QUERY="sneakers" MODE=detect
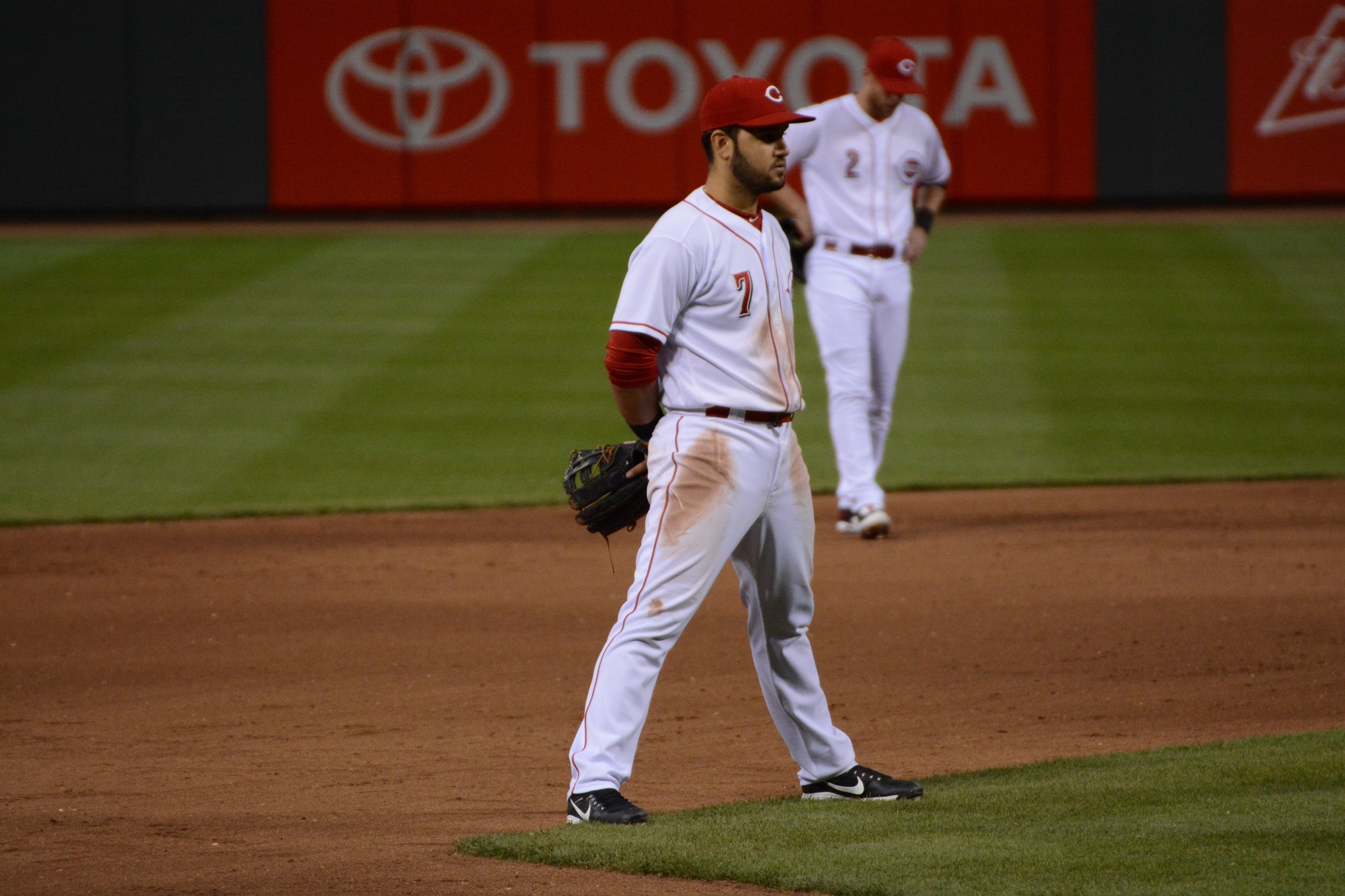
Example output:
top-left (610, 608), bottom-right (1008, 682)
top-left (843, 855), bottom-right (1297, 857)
top-left (837, 509), bottom-right (861, 535)
top-left (859, 504), bottom-right (890, 537)
top-left (801, 765), bottom-right (924, 802)
top-left (566, 788), bottom-right (648, 824)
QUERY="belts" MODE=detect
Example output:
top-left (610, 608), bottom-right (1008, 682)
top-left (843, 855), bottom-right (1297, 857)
top-left (825, 242), bottom-right (895, 259)
top-left (705, 406), bottom-right (793, 427)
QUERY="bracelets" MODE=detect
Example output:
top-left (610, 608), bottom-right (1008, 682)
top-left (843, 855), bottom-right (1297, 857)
top-left (915, 207), bottom-right (933, 232)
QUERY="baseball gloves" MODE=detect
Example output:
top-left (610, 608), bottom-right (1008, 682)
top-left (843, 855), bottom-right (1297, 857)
top-left (776, 217), bottom-right (812, 282)
top-left (562, 439), bottom-right (651, 537)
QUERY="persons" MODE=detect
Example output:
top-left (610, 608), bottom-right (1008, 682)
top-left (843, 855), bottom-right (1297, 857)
top-left (565, 78), bottom-right (924, 825)
top-left (765, 37), bottom-right (953, 535)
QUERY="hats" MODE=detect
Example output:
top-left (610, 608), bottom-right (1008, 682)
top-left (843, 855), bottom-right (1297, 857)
top-left (701, 75), bottom-right (816, 131)
top-left (868, 38), bottom-right (926, 95)
